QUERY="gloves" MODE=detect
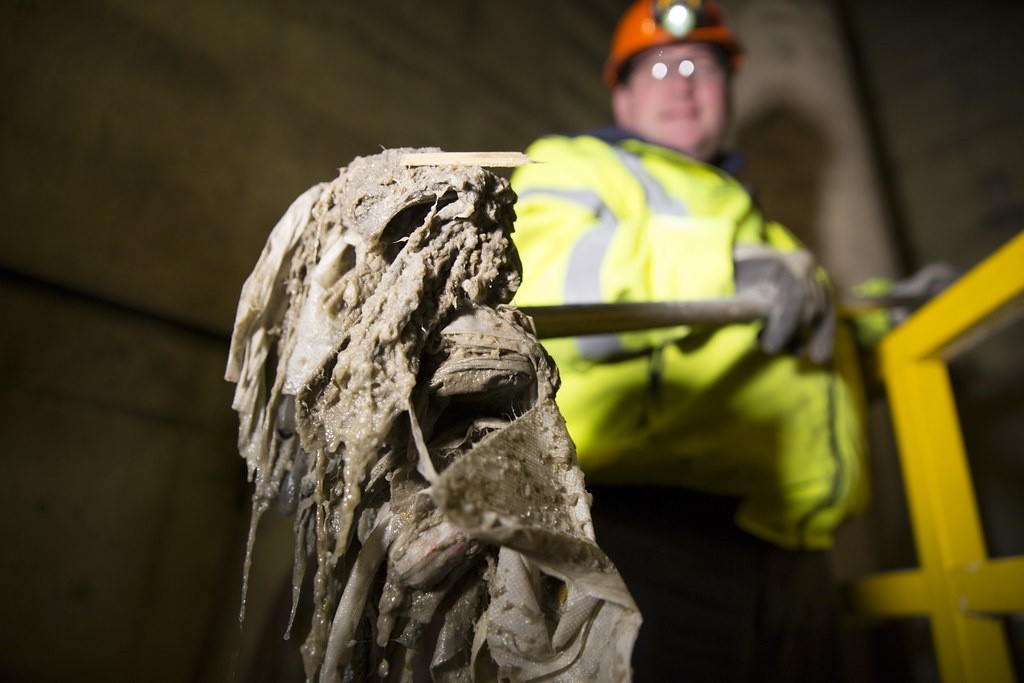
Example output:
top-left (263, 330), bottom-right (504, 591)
top-left (891, 262), bottom-right (954, 322)
top-left (734, 250), bottom-right (838, 365)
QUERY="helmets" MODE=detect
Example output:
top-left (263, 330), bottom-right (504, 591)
top-left (603, 0), bottom-right (746, 90)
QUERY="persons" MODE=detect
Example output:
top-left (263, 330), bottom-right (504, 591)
top-left (503, 1), bottom-right (963, 683)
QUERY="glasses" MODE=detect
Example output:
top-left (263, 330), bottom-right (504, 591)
top-left (631, 56), bottom-right (725, 82)
